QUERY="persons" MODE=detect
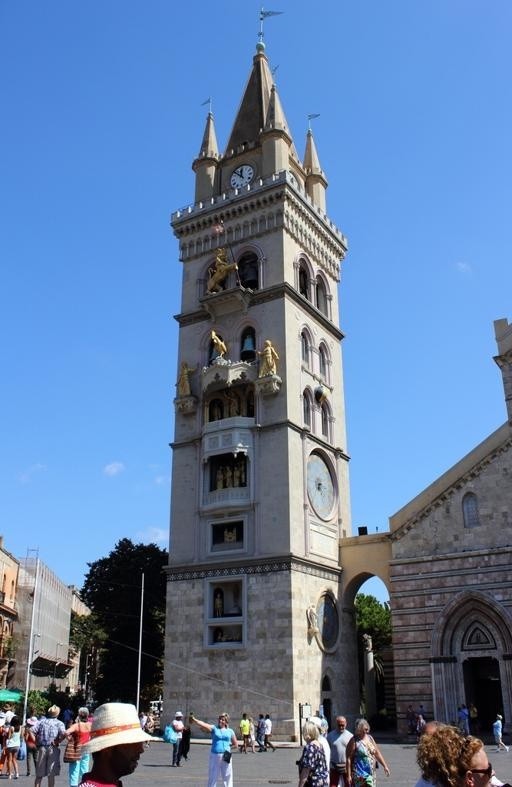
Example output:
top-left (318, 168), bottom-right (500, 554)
top-left (416, 725), bottom-right (511, 786)
top-left (457, 707), bottom-right (462, 726)
top-left (327, 716), bottom-right (355, 787)
top-left (190, 711), bottom-right (238, 787)
top-left (310, 716), bottom-right (332, 785)
top-left (309, 603), bottom-right (319, 628)
top-left (174, 361), bottom-right (199, 397)
top-left (491, 714), bottom-right (509, 752)
top-left (416, 715), bottom-right (427, 736)
top-left (2, 700), bottom-right (92, 787)
top-left (215, 593), bottom-right (222, 617)
top-left (216, 461), bottom-right (245, 489)
top-left (418, 705), bottom-right (425, 717)
top-left (408, 704), bottom-right (415, 727)
top-left (239, 713), bottom-right (276, 754)
top-left (140, 711), bottom-right (147, 728)
top-left (345, 718), bottom-right (390, 787)
top-left (414, 720), bottom-right (450, 787)
top-left (460, 703), bottom-right (470, 733)
top-left (293, 722), bottom-right (330, 786)
top-left (168, 711), bottom-right (188, 767)
top-left (78, 701), bottom-right (165, 786)
top-left (256, 340), bottom-right (278, 379)
top-left (468, 703), bottom-right (478, 732)
top-left (143, 716), bottom-right (155, 748)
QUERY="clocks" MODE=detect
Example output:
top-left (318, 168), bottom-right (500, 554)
top-left (229, 163), bottom-right (254, 188)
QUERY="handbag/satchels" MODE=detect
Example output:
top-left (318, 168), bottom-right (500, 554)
top-left (63, 735), bottom-right (82, 762)
top-left (5, 733), bottom-right (21, 749)
top-left (163, 724), bottom-right (178, 743)
top-left (224, 750), bottom-right (231, 763)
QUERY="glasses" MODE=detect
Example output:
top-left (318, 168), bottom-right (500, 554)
top-left (471, 762), bottom-right (493, 779)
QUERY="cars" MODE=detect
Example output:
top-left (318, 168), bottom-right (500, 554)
top-left (146, 701), bottom-right (164, 715)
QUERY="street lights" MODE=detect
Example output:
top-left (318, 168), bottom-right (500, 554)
top-left (51, 641), bottom-right (68, 707)
top-left (84, 653), bottom-right (95, 705)
top-left (21, 633), bottom-right (42, 742)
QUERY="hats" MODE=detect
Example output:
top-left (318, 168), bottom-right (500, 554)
top-left (3, 703), bottom-right (12, 710)
top-left (25, 704), bottom-right (61, 725)
top-left (78, 700), bottom-right (165, 755)
top-left (174, 712), bottom-right (183, 718)
